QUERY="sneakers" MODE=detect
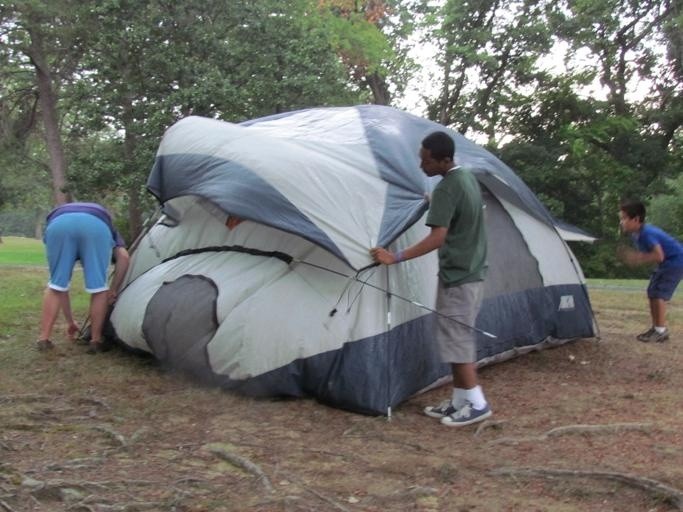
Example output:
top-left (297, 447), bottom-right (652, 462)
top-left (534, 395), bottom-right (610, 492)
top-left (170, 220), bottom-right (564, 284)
top-left (637, 328), bottom-right (668, 342)
top-left (89, 338), bottom-right (112, 353)
top-left (422, 399), bottom-right (456, 419)
top-left (37, 338), bottom-right (55, 350)
top-left (441, 399), bottom-right (493, 426)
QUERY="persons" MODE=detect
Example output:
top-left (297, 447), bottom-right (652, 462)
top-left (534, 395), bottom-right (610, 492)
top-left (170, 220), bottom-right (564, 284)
top-left (369, 129), bottom-right (496, 428)
top-left (38, 200), bottom-right (130, 353)
top-left (616, 198), bottom-right (683, 343)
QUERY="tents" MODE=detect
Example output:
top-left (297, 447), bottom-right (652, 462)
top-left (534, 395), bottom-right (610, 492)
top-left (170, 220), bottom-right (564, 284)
top-left (76, 104), bottom-right (604, 414)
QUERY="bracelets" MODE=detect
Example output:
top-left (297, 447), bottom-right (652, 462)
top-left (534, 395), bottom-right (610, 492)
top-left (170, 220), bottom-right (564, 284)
top-left (394, 250), bottom-right (402, 263)
